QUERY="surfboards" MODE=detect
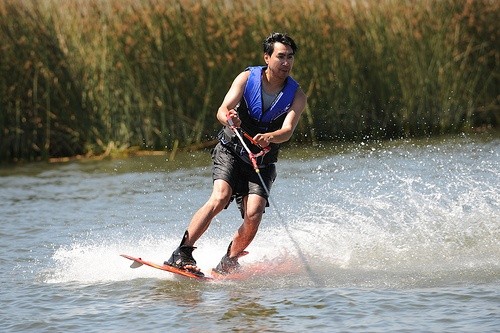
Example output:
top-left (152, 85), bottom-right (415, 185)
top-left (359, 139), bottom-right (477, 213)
top-left (123, 252), bottom-right (258, 282)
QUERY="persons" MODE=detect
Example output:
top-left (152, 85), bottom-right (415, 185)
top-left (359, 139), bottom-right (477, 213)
top-left (163, 32), bottom-right (307, 278)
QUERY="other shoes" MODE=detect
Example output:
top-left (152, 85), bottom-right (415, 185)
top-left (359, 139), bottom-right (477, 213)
top-left (164, 230), bottom-right (205, 278)
top-left (216, 242), bottom-right (249, 278)
